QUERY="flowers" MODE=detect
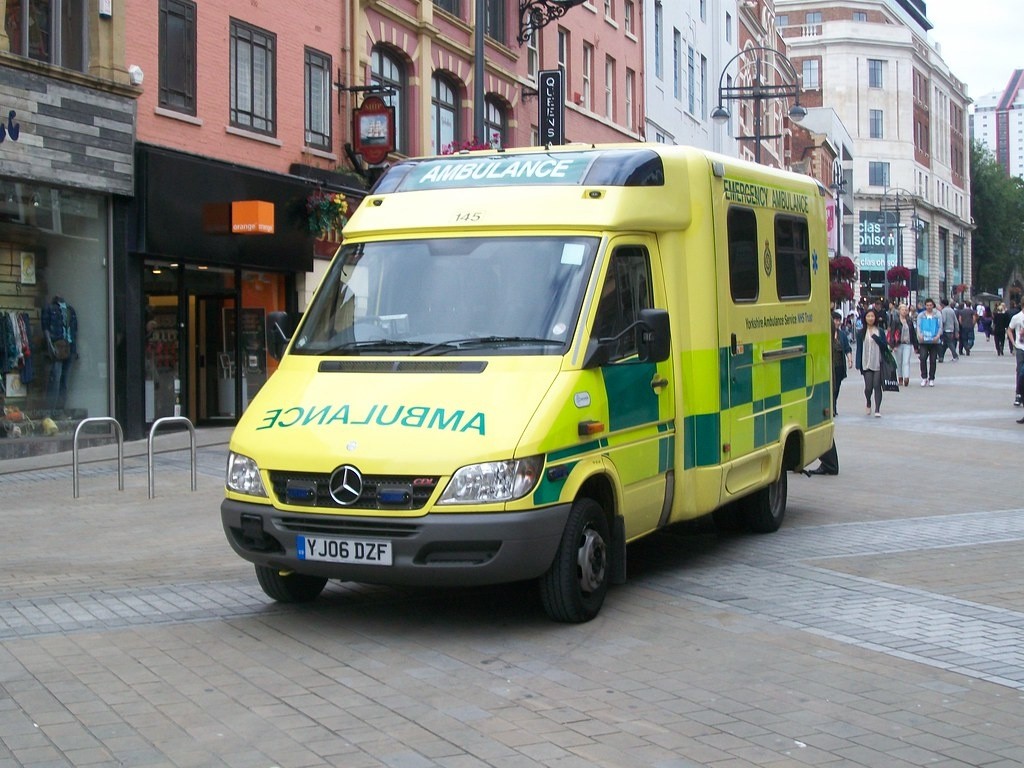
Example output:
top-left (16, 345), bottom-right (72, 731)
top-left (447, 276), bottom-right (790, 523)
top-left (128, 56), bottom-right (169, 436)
top-left (307, 190), bottom-right (348, 235)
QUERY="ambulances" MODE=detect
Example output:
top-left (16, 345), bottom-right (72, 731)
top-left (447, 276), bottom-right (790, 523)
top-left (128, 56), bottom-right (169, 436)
top-left (219, 139), bottom-right (834, 623)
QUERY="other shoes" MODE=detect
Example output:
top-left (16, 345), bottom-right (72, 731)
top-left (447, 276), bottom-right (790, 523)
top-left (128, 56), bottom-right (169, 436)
top-left (952, 357), bottom-right (959, 363)
top-left (866, 407), bottom-right (871, 415)
top-left (965, 346), bottom-right (970, 356)
top-left (874, 413), bottom-right (881, 417)
top-left (1016, 416), bottom-right (1024, 423)
top-left (904, 377), bottom-right (909, 386)
top-left (809, 463), bottom-right (839, 475)
top-left (928, 379), bottom-right (934, 386)
top-left (898, 377), bottom-right (903, 385)
top-left (997, 349), bottom-right (1003, 356)
top-left (938, 357), bottom-right (943, 363)
top-left (920, 378), bottom-right (927, 387)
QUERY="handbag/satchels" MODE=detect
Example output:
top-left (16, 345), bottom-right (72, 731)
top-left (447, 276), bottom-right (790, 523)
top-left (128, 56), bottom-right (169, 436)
top-left (47, 339), bottom-right (71, 363)
top-left (880, 351), bottom-right (899, 392)
top-left (919, 318), bottom-right (941, 344)
top-left (886, 328), bottom-right (900, 346)
top-left (978, 320), bottom-right (985, 332)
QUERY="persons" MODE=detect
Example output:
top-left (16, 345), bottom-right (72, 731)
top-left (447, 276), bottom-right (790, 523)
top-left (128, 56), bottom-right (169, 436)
top-left (830, 296), bottom-right (1024, 424)
top-left (41, 295), bottom-right (78, 419)
top-left (593, 270), bottom-right (619, 338)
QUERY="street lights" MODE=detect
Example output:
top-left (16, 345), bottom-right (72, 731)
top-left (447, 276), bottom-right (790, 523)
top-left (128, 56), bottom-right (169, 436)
top-left (953, 226), bottom-right (968, 300)
top-left (828, 160), bottom-right (848, 256)
top-left (878, 188), bottom-right (918, 266)
top-left (709, 47), bottom-right (808, 164)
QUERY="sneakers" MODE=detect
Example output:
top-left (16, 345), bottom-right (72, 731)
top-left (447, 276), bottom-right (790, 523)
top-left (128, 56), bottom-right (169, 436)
top-left (1014, 393), bottom-right (1022, 405)
